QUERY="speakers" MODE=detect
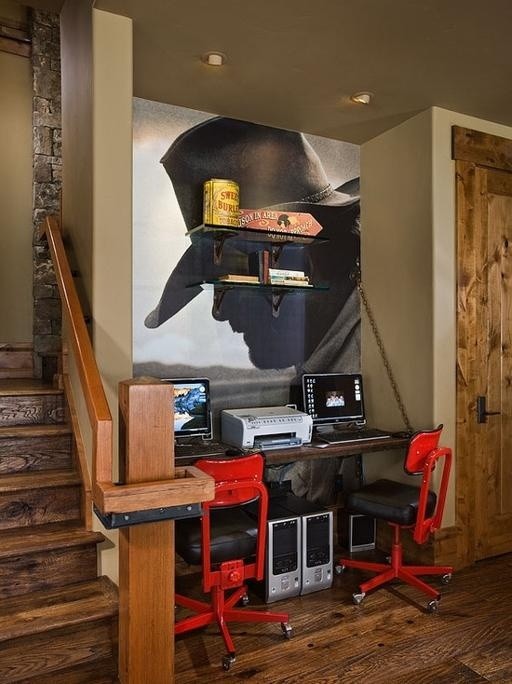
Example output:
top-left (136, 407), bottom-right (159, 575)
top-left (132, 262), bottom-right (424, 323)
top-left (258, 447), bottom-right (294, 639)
top-left (337, 508), bottom-right (377, 553)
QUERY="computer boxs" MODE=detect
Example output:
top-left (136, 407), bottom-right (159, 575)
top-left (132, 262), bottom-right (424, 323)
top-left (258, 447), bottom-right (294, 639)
top-left (241, 494), bottom-right (333, 604)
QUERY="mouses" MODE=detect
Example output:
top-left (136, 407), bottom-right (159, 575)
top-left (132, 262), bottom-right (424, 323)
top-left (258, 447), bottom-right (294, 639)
top-left (392, 431), bottom-right (410, 439)
top-left (224, 448), bottom-right (240, 456)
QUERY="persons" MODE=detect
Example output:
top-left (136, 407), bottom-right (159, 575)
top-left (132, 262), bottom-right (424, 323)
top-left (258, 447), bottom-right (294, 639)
top-left (326, 394), bottom-right (345, 407)
top-left (144, 116), bottom-right (360, 412)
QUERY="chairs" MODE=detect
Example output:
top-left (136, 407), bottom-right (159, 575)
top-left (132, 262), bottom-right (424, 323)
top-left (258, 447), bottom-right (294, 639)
top-left (172, 453), bottom-right (292, 673)
top-left (334, 424), bottom-right (463, 615)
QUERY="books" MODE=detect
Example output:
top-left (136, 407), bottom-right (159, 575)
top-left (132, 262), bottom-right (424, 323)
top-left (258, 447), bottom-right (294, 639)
top-left (218, 250), bottom-right (314, 287)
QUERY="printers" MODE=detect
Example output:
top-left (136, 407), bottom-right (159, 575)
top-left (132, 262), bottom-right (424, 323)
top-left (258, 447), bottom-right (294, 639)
top-left (220, 406), bottom-right (313, 450)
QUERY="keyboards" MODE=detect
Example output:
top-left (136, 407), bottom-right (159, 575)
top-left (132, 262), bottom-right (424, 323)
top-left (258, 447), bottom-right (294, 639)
top-left (317, 428), bottom-right (391, 444)
top-left (174, 444), bottom-right (225, 459)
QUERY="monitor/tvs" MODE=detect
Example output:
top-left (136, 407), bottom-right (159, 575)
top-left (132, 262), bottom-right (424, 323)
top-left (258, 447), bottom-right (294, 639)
top-left (302, 373), bottom-right (367, 434)
top-left (161, 377), bottom-right (213, 445)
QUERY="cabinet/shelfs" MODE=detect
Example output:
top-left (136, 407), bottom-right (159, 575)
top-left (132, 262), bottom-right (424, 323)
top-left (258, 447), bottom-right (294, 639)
top-left (183, 220), bottom-right (330, 318)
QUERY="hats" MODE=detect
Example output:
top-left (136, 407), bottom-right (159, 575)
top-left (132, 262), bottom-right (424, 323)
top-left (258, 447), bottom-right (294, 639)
top-left (144, 116), bottom-right (361, 329)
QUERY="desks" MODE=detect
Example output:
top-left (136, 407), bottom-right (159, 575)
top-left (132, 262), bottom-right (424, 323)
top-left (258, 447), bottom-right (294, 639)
top-left (170, 426), bottom-right (413, 471)
